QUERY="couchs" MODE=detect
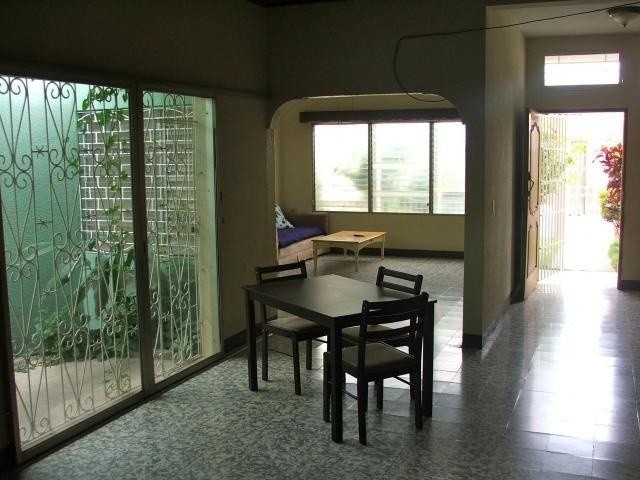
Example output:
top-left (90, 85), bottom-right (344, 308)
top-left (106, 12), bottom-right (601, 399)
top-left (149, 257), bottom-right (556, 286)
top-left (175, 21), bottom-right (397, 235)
top-left (273, 212), bottom-right (330, 267)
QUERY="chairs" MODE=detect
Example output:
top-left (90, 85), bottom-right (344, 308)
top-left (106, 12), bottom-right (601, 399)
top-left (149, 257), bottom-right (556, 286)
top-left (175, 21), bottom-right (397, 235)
top-left (323, 292), bottom-right (429, 445)
top-left (255, 260), bottom-right (330, 396)
top-left (341, 266), bottom-right (423, 409)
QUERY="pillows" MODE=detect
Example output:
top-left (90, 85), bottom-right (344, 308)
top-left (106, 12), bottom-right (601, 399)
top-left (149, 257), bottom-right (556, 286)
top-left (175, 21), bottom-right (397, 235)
top-left (275, 203), bottom-right (295, 229)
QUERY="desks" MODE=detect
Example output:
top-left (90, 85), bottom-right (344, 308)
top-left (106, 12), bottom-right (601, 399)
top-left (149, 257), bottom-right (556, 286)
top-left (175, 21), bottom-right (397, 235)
top-left (311, 230), bottom-right (387, 273)
top-left (241, 274), bottom-right (437, 443)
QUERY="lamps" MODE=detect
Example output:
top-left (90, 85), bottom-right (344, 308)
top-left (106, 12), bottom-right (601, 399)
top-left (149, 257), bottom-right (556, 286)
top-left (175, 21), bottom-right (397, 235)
top-left (608, 6), bottom-right (640, 28)
